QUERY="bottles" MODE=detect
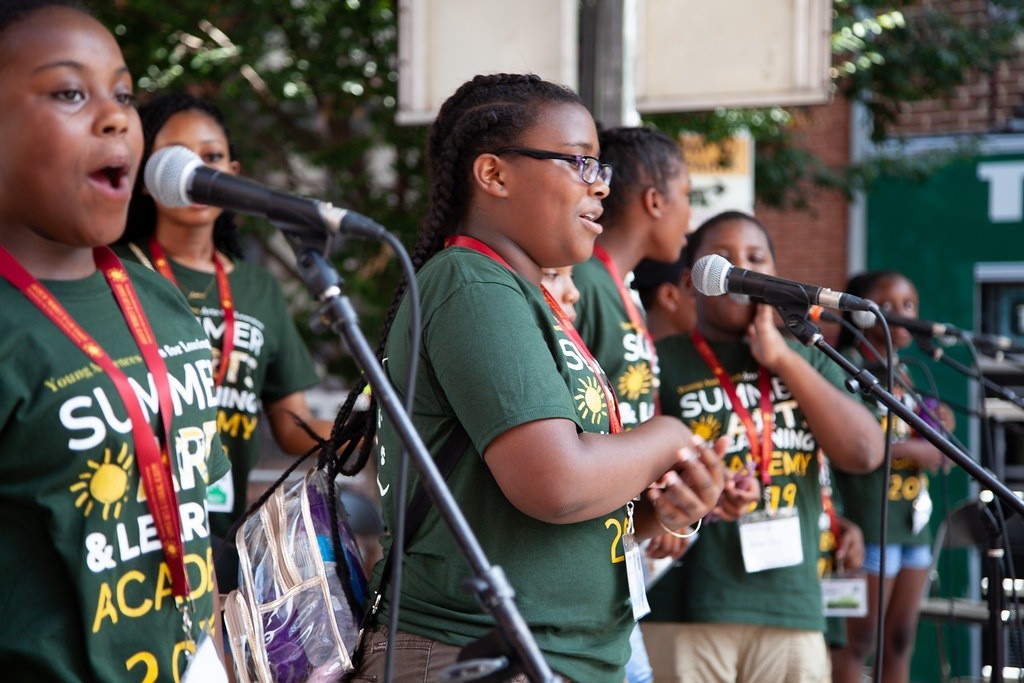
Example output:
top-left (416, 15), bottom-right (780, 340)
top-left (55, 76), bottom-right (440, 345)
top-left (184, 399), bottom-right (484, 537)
top-left (911, 395), bottom-right (941, 439)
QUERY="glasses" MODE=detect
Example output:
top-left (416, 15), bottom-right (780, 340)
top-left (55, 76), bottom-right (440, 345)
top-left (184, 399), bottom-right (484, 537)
top-left (492, 146), bottom-right (613, 186)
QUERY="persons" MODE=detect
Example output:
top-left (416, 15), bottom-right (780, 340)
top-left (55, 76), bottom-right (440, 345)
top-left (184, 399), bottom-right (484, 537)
top-left (0, 0), bottom-right (220, 683)
top-left (345, 73), bottom-right (730, 683)
top-left (570, 128), bottom-right (692, 430)
top-left (631, 211), bottom-right (886, 683)
top-left (107, 93), bottom-right (363, 593)
top-left (832, 272), bottom-right (953, 683)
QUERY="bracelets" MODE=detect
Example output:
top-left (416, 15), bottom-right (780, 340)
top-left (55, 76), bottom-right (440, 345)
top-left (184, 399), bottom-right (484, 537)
top-left (655, 516), bottom-right (702, 538)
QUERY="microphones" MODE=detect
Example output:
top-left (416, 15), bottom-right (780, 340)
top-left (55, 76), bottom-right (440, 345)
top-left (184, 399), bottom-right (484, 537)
top-left (691, 253), bottom-right (1024, 356)
top-left (144, 146), bottom-right (385, 243)
